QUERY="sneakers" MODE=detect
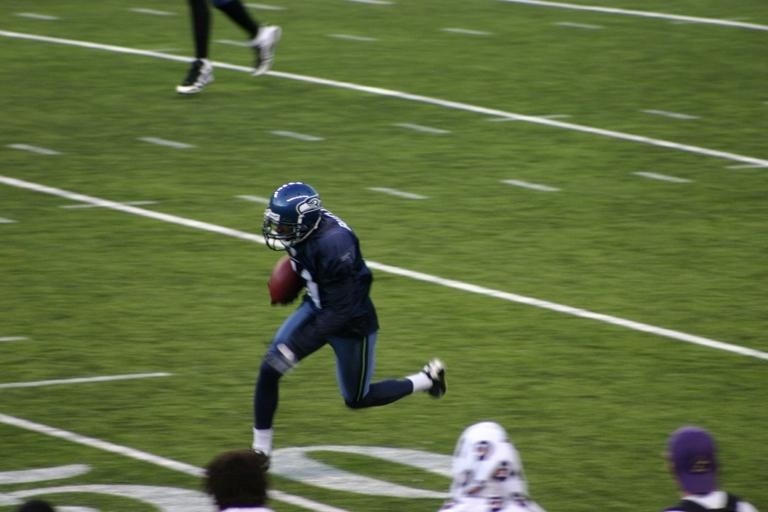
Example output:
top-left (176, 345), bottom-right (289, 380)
top-left (422, 358), bottom-right (446, 398)
top-left (176, 59), bottom-right (214, 94)
top-left (253, 25), bottom-right (280, 76)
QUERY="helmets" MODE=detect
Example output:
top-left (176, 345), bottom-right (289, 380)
top-left (262, 182), bottom-right (321, 251)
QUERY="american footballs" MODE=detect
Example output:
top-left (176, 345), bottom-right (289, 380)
top-left (269, 255), bottom-right (303, 305)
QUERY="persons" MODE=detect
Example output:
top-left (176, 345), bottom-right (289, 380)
top-left (18, 501), bottom-right (53, 512)
top-left (252, 182), bottom-right (446, 456)
top-left (176, 0), bottom-right (282, 94)
top-left (661, 427), bottom-right (761, 512)
top-left (203, 449), bottom-right (272, 512)
top-left (438, 422), bottom-right (547, 512)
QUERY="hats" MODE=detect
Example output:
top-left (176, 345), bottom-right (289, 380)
top-left (669, 426), bottom-right (718, 494)
